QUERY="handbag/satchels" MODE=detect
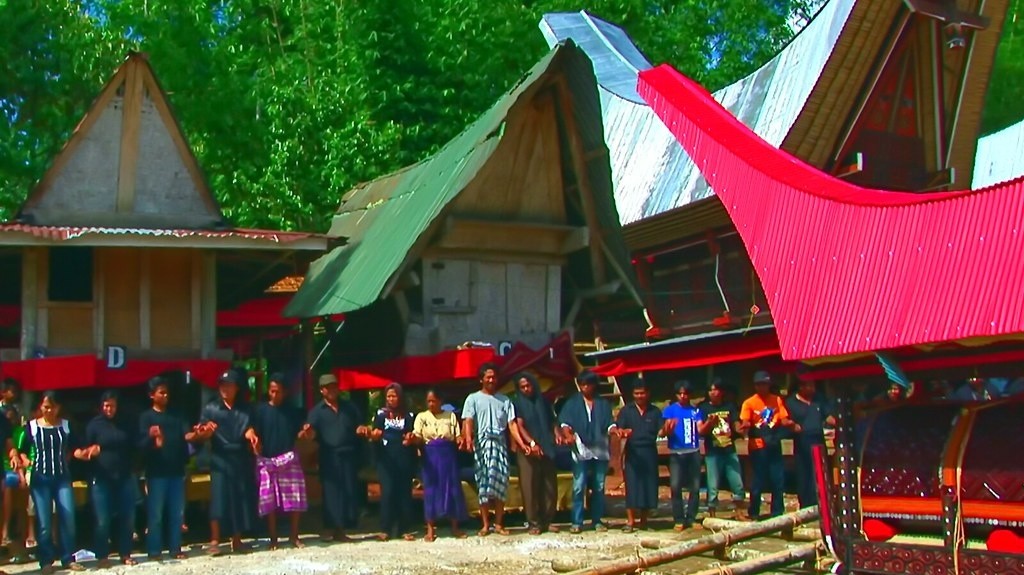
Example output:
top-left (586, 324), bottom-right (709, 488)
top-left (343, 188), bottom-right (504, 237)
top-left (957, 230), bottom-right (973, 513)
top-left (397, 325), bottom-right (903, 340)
top-left (712, 415), bottom-right (733, 448)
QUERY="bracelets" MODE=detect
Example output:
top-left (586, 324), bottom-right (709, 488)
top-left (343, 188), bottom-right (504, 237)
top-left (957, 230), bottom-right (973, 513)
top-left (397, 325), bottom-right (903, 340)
top-left (528, 440), bottom-right (538, 448)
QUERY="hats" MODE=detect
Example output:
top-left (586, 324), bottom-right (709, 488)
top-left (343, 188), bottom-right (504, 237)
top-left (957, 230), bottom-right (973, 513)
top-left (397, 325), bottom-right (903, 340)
top-left (145, 376), bottom-right (168, 394)
top-left (319, 374), bottom-right (337, 387)
top-left (219, 371), bottom-right (241, 385)
top-left (754, 371), bottom-right (771, 384)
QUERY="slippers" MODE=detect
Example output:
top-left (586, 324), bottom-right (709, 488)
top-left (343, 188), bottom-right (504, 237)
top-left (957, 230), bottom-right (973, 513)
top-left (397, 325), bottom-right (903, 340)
top-left (97, 526), bottom-right (469, 570)
top-left (41, 562), bottom-right (53, 574)
top-left (26, 539), bottom-right (37, 547)
top-left (1, 535), bottom-right (12, 546)
top-left (478, 514), bottom-right (752, 539)
top-left (64, 561), bottom-right (87, 571)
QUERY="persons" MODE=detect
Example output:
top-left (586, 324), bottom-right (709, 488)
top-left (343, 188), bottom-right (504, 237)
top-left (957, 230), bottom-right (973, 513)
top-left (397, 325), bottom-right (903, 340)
top-left (696, 378), bottom-right (748, 525)
top-left (618, 378), bottom-right (669, 532)
top-left (200, 369), bottom-right (261, 557)
top-left (511, 372), bottom-right (565, 536)
top-left (738, 369), bottom-right (794, 521)
top-left (303, 375), bottom-right (370, 543)
top-left (1, 376), bottom-right (59, 564)
top-left (787, 376), bottom-right (839, 508)
top-left (558, 371), bottom-right (626, 534)
top-left (883, 379), bottom-right (906, 404)
top-left (84, 392), bottom-right (162, 571)
top-left (370, 382), bottom-right (419, 542)
top-left (661, 378), bottom-right (717, 533)
top-left (461, 361), bottom-right (531, 536)
top-left (957, 377), bottom-right (1001, 401)
top-left (20, 391), bottom-right (92, 574)
top-left (405, 388), bottom-right (469, 543)
top-left (141, 376), bottom-right (209, 561)
top-left (249, 378), bottom-right (313, 551)
